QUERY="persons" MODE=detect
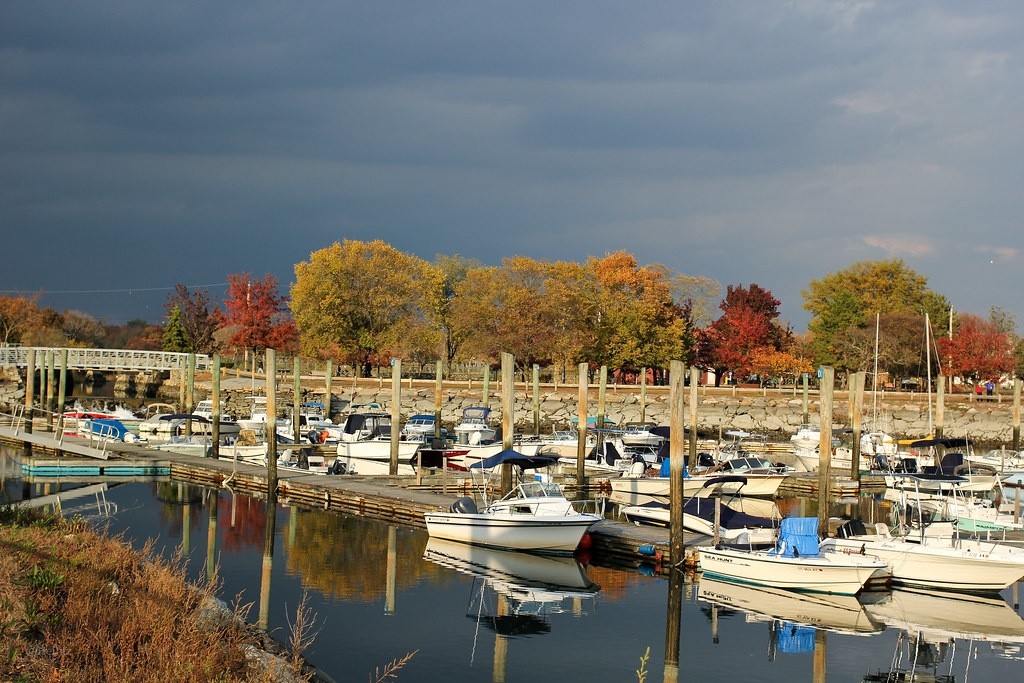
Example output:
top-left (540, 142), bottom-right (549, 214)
top-left (336, 360), bottom-right (372, 378)
top-left (976, 379), bottom-right (994, 402)
top-left (259, 360), bottom-right (264, 373)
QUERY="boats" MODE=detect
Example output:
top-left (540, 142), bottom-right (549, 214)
top-left (50, 391), bottom-right (1024, 639)
top-left (423, 433), bottom-right (605, 552)
top-left (422, 534), bottom-right (602, 664)
